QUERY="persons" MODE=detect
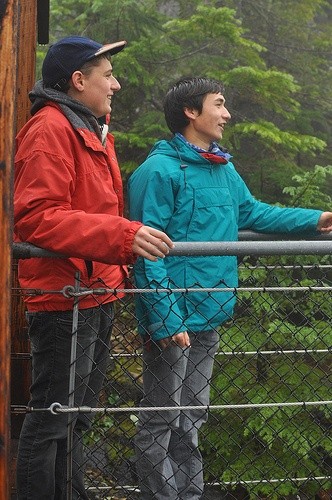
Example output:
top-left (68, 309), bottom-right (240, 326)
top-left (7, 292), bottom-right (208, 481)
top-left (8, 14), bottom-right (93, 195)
top-left (126, 76), bottom-right (332, 500)
top-left (13, 36), bottom-right (175, 500)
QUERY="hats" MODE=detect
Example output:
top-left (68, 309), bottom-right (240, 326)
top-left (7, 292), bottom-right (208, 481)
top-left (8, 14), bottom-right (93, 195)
top-left (41, 37), bottom-right (127, 91)
